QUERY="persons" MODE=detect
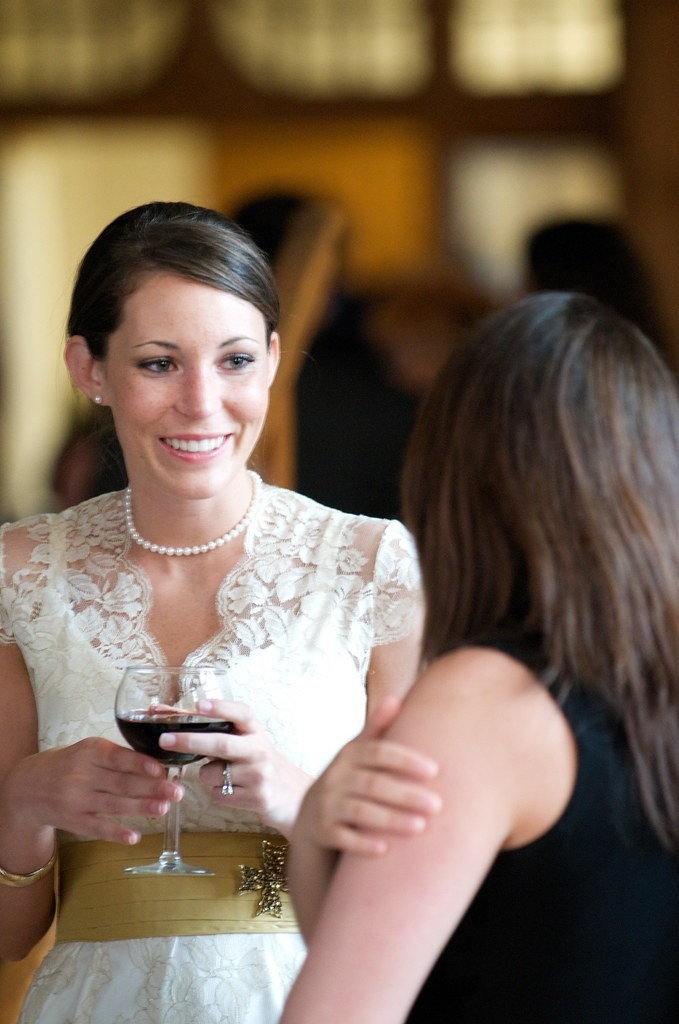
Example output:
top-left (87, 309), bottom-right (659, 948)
top-left (522, 215), bottom-right (665, 352)
top-left (224, 186), bottom-right (429, 514)
top-left (282, 292), bottom-right (679, 1024)
top-left (0, 198), bottom-right (429, 1024)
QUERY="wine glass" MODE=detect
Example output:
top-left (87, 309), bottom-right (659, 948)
top-left (114, 666), bottom-right (234, 875)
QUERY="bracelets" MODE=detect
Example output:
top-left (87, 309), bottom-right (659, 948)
top-left (1, 841), bottom-right (59, 887)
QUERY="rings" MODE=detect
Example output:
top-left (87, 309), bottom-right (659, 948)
top-left (220, 761), bottom-right (234, 796)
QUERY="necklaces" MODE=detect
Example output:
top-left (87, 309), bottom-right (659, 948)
top-left (124, 469), bottom-right (262, 555)
